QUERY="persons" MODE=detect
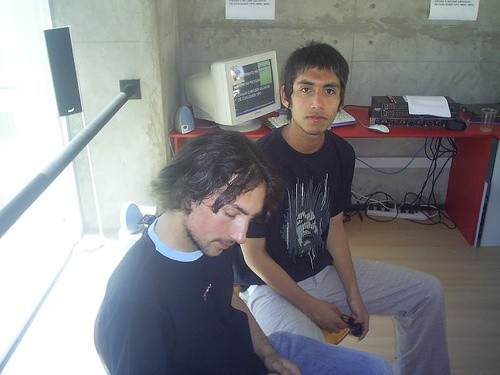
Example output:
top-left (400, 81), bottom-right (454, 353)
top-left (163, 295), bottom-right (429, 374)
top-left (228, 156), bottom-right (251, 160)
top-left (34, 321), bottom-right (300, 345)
top-left (231, 40), bottom-right (451, 375)
top-left (96, 129), bottom-right (305, 375)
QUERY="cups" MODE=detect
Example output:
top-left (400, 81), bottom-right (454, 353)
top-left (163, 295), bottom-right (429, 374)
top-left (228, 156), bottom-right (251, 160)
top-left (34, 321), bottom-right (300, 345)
top-left (479, 107), bottom-right (498, 132)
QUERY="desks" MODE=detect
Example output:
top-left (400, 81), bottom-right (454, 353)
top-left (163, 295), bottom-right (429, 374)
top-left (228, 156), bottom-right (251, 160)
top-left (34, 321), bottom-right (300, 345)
top-left (169, 104), bottom-right (500, 247)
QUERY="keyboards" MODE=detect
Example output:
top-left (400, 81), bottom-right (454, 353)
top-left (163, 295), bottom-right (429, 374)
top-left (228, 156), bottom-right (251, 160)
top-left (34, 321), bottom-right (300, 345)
top-left (265, 108), bottom-right (357, 131)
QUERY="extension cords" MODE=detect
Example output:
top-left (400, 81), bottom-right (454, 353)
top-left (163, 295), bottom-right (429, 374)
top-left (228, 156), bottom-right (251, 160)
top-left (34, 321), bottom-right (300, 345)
top-left (367, 206), bottom-right (428, 220)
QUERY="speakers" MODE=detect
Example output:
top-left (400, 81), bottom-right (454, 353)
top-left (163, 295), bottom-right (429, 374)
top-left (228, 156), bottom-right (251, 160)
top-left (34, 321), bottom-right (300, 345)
top-left (174, 106), bottom-right (194, 134)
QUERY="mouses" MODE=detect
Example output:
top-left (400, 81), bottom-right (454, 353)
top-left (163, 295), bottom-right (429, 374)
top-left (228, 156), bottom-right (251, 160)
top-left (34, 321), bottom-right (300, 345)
top-left (368, 124), bottom-right (389, 134)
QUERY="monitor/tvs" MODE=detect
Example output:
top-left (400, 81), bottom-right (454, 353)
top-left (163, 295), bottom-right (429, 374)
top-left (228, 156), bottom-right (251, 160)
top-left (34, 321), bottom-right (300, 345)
top-left (185, 51), bottom-right (281, 132)
